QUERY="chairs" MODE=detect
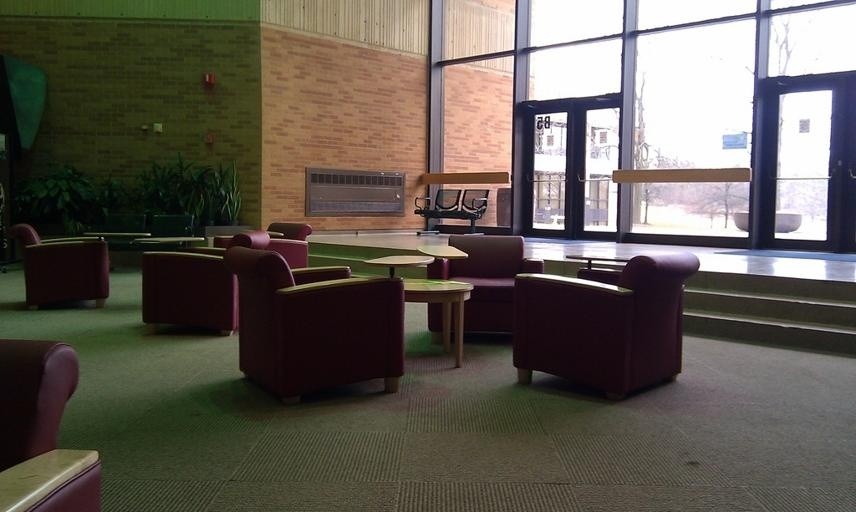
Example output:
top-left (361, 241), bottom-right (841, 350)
top-left (1, 338), bottom-right (100, 511)
top-left (142, 230), bottom-right (269, 336)
top-left (14, 223), bottom-right (109, 310)
top-left (511, 251), bottom-right (700, 401)
top-left (83, 213), bottom-right (312, 268)
top-left (414, 236), bottom-right (544, 342)
top-left (226, 245), bottom-right (405, 404)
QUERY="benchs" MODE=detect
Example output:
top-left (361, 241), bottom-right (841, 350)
top-left (413, 188), bottom-right (490, 236)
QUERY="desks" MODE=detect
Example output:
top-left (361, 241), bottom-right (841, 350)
top-left (350, 256), bottom-right (474, 368)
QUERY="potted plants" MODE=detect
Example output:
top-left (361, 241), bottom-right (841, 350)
top-left (1, 188), bottom-right (240, 261)
